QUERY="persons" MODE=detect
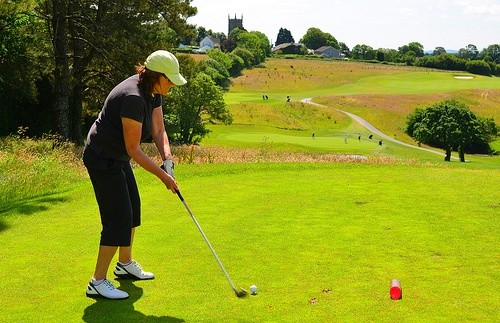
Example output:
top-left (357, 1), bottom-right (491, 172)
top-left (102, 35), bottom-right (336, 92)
top-left (82, 50), bottom-right (188, 300)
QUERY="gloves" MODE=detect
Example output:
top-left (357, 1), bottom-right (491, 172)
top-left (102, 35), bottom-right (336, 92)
top-left (161, 159), bottom-right (174, 177)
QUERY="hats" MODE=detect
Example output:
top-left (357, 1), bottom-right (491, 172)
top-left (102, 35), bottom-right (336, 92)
top-left (144, 50), bottom-right (187, 86)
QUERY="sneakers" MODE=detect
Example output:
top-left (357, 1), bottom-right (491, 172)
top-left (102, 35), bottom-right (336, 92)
top-left (86, 279), bottom-right (129, 299)
top-left (113, 260), bottom-right (154, 279)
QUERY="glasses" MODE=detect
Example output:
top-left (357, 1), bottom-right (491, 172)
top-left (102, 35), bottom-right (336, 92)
top-left (162, 75), bottom-right (174, 86)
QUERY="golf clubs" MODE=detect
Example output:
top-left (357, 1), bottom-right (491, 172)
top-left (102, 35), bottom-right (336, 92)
top-left (160, 165), bottom-right (248, 298)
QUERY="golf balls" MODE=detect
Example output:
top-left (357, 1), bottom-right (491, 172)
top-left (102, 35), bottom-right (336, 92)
top-left (250, 286), bottom-right (257, 292)
